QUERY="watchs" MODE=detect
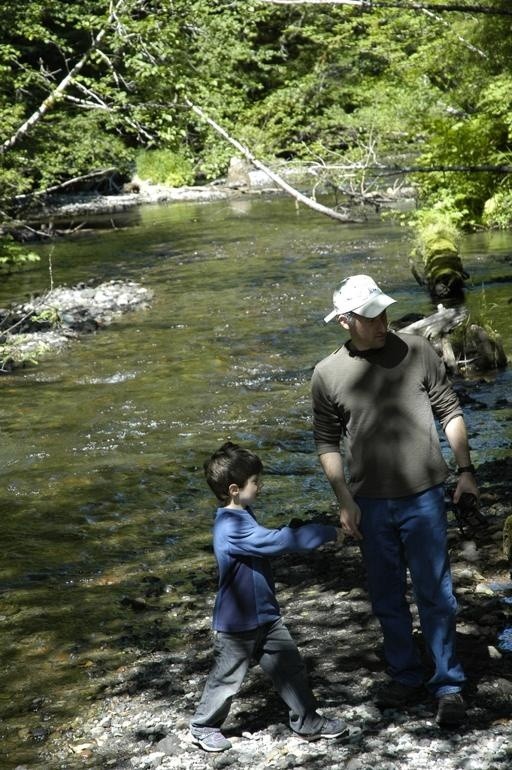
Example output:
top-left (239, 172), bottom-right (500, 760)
top-left (458, 463), bottom-right (476, 474)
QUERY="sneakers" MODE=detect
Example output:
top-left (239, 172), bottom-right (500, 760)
top-left (292, 718), bottom-right (347, 740)
top-left (190, 724), bottom-right (232, 752)
top-left (378, 682), bottom-right (465, 725)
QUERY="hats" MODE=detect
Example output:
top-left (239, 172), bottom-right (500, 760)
top-left (324, 275), bottom-right (398, 323)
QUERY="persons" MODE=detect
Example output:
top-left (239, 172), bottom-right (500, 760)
top-left (187, 440), bottom-right (354, 753)
top-left (309, 273), bottom-right (483, 726)
